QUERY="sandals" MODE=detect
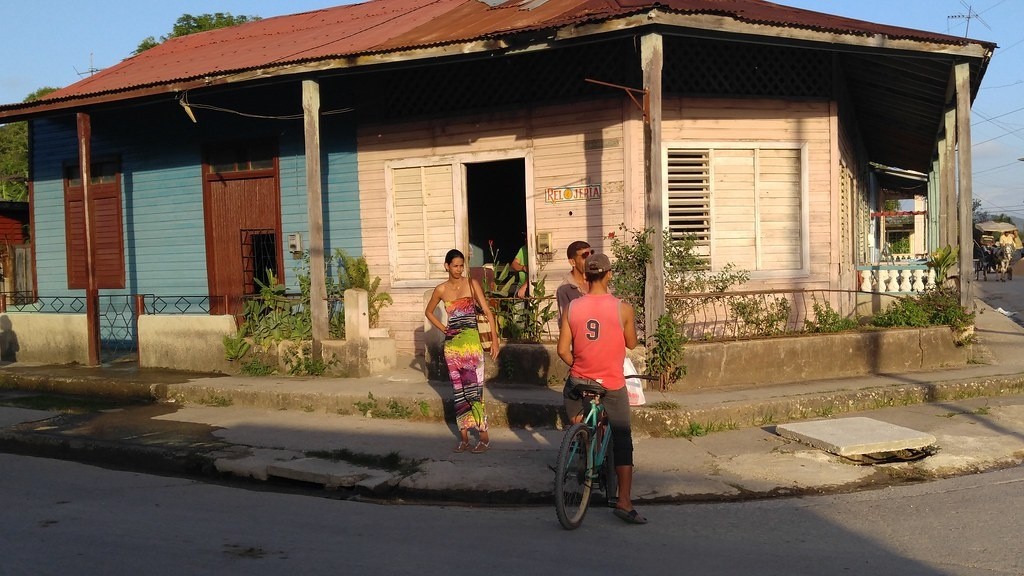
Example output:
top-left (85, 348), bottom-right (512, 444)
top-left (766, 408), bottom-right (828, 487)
top-left (472, 440), bottom-right (490, 453)
top-left (455, 441), bottom-right (470, 453)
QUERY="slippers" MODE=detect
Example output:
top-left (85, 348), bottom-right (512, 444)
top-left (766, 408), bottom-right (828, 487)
top-left (614, 506), bottom-right (648, 523)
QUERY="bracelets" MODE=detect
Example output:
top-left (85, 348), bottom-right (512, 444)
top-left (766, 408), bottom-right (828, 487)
top-left (522, 266), bottom-right (524, 270)
top-left (458, 287), bottom-right (459, 289)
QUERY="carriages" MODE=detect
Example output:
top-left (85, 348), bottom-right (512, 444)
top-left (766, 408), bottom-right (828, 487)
top-left (972, 220), bottom-right (1018, 283)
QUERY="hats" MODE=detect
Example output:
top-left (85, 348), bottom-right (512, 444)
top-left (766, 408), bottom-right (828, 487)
top-left (585, 254), bottom-right (611, 273)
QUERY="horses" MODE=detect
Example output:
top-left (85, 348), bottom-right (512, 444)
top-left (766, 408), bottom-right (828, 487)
top-left (982, 242), bottom-right (1015, 283)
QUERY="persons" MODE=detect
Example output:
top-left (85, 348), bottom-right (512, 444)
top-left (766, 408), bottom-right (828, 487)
top-left (980, 223), bottom-right (1023, 254)
top-left (512, 229), bottom-right (529, 298)
top-left (557, 254), bottom-right (648, 523)
top-left (556, 241), bottom-right (611, 360)
top-left (425, 249), bottom-right (500, 453)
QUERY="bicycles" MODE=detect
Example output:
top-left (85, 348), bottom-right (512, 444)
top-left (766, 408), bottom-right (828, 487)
top-left (552, 375), bottom-right (661, 530)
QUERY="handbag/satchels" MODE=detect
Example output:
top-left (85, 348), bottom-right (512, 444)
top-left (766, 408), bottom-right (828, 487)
top-left (623, 358), bottom-right (645, 406)
top-left (468, 277), bottom-right (500, 352)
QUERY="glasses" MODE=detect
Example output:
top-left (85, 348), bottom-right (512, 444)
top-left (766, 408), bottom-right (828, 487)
top-left (578, 250), bottom-right (595, 258)
